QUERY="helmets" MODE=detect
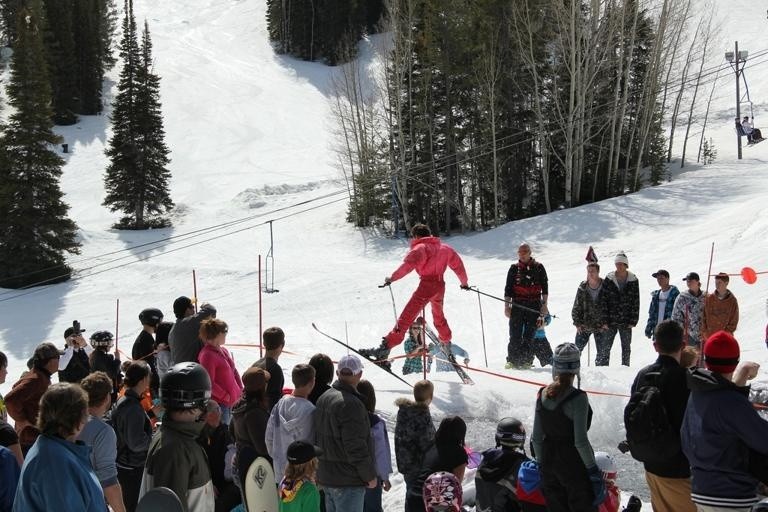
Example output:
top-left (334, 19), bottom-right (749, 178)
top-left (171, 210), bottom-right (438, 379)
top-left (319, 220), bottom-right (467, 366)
top-left (594, 452), bottom-right (618, 485)
top-left (495, 417), bottom-right (526, 449)
top-left (422, 470), bottom-right (462, 511)
top-left (159, 361), bottom-right (211, 413)
top-left (138, 307), bottom-right (163, 328)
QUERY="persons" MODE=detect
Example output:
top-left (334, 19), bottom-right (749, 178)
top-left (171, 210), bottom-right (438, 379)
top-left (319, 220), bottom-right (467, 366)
top-left (734, 116), bottom-right (762, 144)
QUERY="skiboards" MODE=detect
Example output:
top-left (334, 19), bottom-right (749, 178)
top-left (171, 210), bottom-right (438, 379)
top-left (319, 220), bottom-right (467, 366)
top-left (312, 322), bottom-right (475, 389)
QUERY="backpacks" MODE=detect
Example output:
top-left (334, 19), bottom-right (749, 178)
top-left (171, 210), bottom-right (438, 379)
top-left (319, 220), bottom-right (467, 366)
top-left (623, 364), bottom-right (680, 464)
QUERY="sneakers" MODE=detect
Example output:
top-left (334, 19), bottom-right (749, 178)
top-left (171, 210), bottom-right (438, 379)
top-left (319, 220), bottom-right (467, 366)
top-left (365, 347), bottom-right (389, 362)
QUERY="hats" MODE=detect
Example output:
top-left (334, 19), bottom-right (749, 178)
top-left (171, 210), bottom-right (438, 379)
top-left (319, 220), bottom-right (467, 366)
top-left (703, 331), bottom-right (740, 374)
top-left (287, 441), bottom-right (325, 465)
top-left (33, 327), bottom-right (113, 361)
top-left (682, 272), bottom-right (699, 281)
top-left (552, 342), bottom-right (581, 390)
top-left (173, 296), bottom-right (197, 313)
top-left (337, 355), bottom-right (364, 376)
top-left (242, 367), bottom-right (272, 393)
top-left (652, 270), bottom-right (669, 279)
top-left (614, 250), bottom-right (628, 267)
